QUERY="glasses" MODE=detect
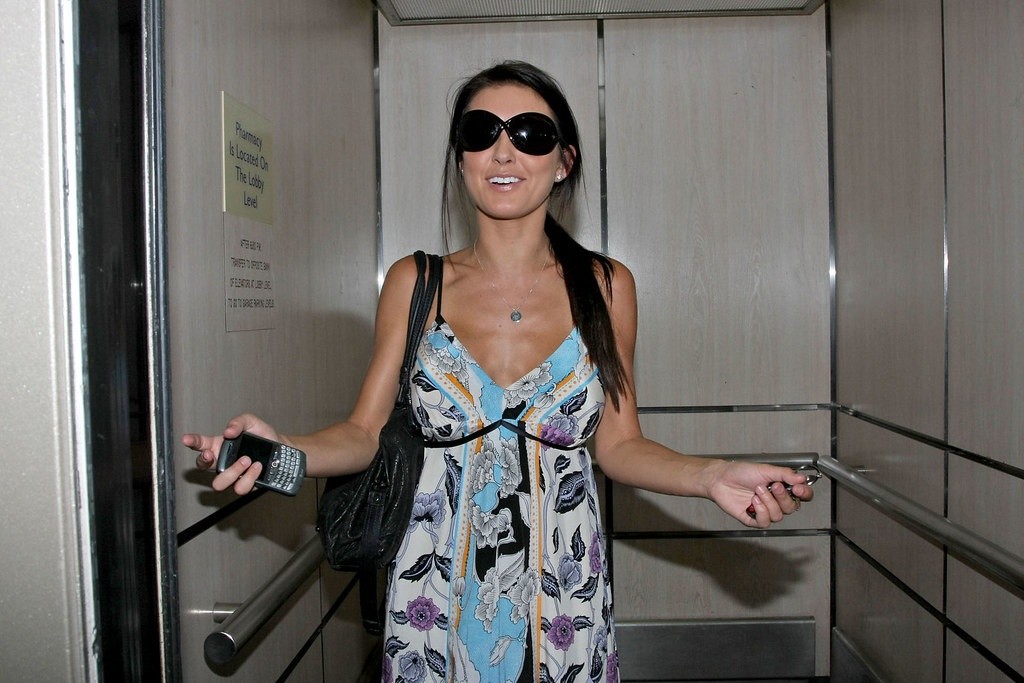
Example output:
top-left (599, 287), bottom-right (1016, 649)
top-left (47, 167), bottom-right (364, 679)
top-left (450, 110), bottom-right (566, 157)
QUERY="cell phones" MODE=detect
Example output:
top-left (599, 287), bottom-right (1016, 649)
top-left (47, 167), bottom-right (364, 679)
top-left (217, 430), bottom-right (306, 495)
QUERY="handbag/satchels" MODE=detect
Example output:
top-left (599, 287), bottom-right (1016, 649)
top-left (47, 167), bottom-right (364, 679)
top-left (315, 250), bottom-right (440, 572)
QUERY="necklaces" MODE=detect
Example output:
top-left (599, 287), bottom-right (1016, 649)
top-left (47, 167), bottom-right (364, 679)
top-left (473, 238), bottom-right (550, 322)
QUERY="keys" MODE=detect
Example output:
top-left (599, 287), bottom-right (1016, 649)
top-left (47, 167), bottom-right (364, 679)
top-left (747, 466), bottom-right (821, 517)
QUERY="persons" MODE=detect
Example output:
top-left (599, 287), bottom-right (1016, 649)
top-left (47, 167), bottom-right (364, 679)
top-left (183, 62), bottom-right (813, 683)
